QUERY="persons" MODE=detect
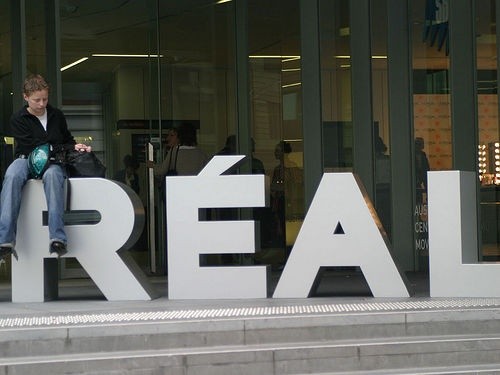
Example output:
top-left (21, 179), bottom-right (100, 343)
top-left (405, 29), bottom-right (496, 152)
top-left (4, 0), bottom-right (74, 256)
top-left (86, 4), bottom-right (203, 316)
top-left (115, 127), bottom-right (432, 256)
top-left (0, 73), bottom-right (91, 261)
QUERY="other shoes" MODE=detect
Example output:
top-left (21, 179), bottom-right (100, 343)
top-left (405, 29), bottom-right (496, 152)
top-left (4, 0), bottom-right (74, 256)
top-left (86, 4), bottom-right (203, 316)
top-left (0, 247), bottom-right (14, 257)
top-left (49, 241), bottom-right (68, 256)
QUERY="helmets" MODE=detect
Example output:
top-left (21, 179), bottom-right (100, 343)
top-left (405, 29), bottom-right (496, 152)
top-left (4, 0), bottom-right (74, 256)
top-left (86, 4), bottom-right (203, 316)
top-left (28, 143), bottom-right (53, 179)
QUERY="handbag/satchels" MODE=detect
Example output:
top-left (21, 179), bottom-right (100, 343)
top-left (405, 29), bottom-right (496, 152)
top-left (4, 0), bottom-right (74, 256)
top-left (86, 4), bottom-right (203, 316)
top-left (165, 144), bottom-right (180, 176)
top-left (67, 150), bottom-right (106, 178)
